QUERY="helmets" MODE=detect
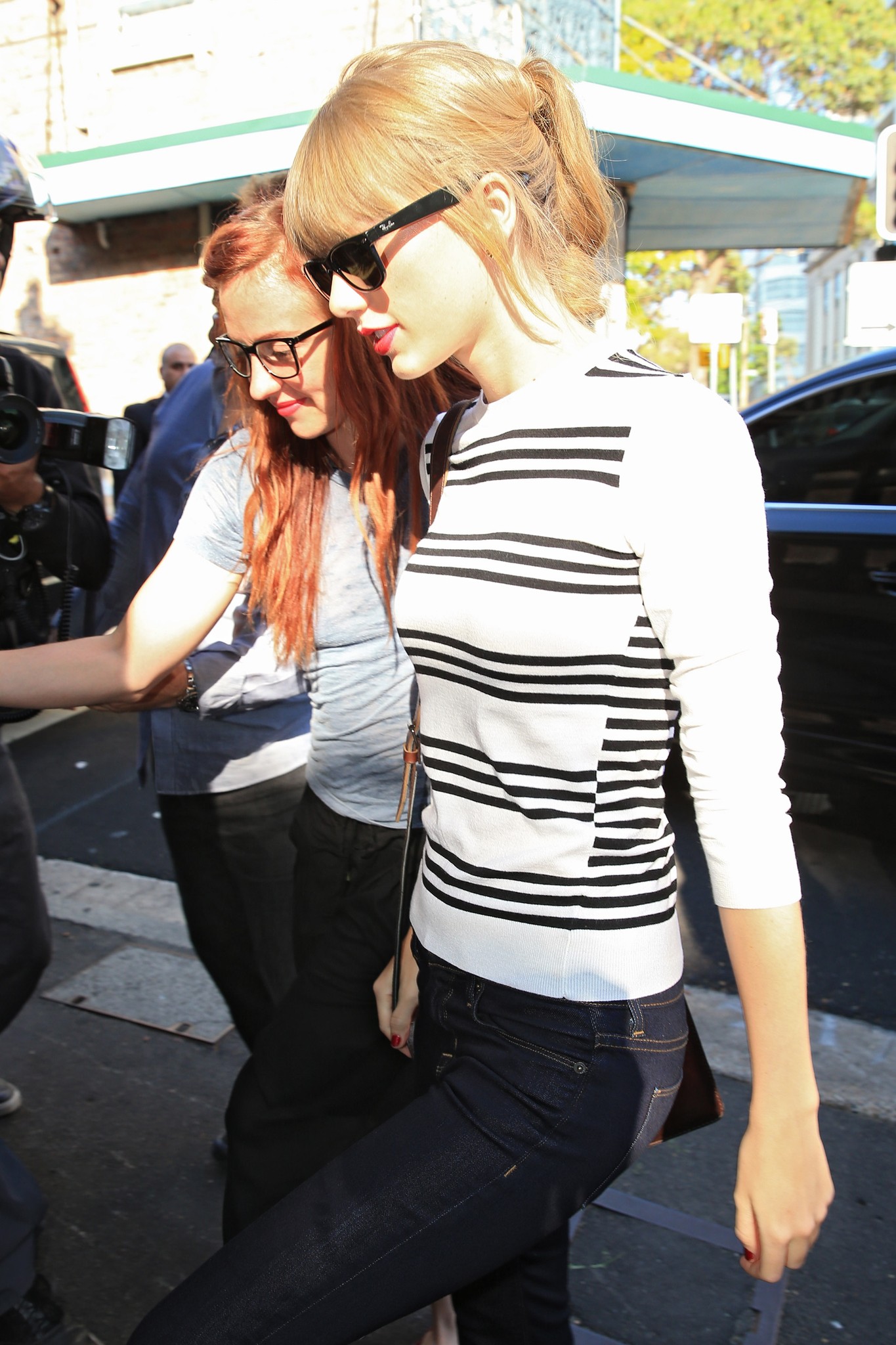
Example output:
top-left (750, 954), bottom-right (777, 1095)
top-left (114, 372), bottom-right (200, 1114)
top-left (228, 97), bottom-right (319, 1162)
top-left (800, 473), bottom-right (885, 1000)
top-left (0, 138), bottom-right (55, 221)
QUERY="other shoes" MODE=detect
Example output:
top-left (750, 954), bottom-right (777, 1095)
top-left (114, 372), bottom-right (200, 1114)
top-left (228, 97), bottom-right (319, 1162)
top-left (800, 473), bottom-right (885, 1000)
top-left (0, 1081), bottom-right (21, 1114)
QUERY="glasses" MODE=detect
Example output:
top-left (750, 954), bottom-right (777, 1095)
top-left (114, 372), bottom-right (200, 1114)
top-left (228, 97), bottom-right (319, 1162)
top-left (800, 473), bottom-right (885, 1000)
top-left (216, 318), bottom-right (339, 377)
top-left (302, 172), bottom-right (532, 305)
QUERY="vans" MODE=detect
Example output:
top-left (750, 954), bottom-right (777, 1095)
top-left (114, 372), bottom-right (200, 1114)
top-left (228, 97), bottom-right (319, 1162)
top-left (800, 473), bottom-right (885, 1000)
top-left (0, 332), bottom-right (109, 503)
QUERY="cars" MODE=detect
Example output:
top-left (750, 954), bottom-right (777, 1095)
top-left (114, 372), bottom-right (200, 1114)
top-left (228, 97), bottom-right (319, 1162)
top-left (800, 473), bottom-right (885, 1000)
top-left (735, 345), bottom-right (896, 787)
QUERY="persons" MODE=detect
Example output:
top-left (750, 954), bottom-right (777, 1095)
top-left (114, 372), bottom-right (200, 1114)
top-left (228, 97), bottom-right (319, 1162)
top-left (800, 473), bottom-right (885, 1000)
top-left (0, 143), bottom-right (480, 1345)
top-left (133, 38), bottom-right (841, 1345)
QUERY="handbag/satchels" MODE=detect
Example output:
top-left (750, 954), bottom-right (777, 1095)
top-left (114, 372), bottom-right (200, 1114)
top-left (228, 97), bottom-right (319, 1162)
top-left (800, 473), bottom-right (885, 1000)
top-left (652, 1003), bottom-right (723, 1143)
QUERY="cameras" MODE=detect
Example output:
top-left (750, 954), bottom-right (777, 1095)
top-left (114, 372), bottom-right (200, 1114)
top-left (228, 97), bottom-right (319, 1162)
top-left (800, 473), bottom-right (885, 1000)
top-left (0, 392), bottom-right (137, 472)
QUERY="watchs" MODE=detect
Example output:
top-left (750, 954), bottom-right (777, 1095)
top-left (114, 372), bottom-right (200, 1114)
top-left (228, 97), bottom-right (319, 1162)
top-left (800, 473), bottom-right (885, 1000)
top-left (176, 659), bottom-right (201, 712)
